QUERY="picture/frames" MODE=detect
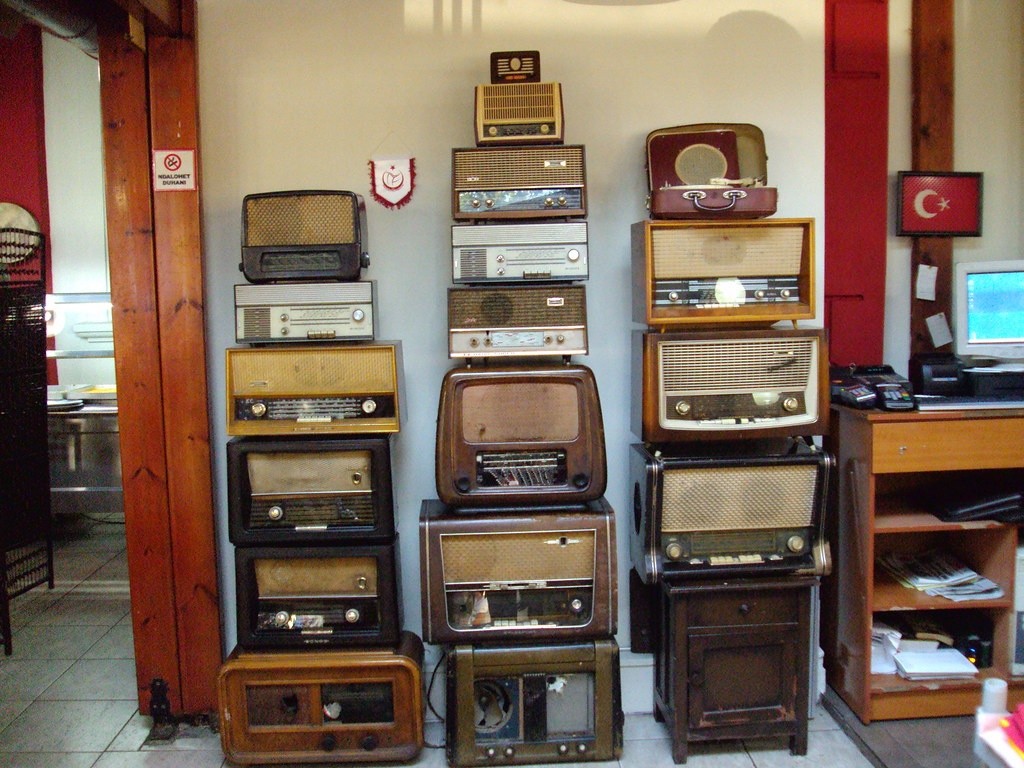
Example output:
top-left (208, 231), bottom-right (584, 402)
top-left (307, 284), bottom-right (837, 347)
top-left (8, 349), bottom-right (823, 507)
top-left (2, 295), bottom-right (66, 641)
top-left (896, 169), bottom-right (984, 239)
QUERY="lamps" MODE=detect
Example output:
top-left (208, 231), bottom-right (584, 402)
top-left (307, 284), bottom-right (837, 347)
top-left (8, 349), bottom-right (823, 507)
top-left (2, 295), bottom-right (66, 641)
top-left (25, 305), bottom-right (64, 338)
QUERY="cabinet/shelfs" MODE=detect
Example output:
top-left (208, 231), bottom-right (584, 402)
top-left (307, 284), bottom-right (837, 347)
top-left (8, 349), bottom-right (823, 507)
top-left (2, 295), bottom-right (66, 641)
top-left (651, 574), bottom-right (820, 765)
top-left (825, 395), bottom-right (1024, 727)
top-left (0, 227), bottom-right (55, 656)
top-left (45, 293), bottom-right (125, 515)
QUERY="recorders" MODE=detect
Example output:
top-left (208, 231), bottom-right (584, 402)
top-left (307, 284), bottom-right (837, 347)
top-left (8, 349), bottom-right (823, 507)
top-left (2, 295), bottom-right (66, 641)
top-left (218, 50), bottom-right (832, 766)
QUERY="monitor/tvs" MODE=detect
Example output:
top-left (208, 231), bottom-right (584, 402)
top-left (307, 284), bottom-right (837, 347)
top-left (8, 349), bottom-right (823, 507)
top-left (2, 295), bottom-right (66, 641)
top-left (956, 259), bottom-right (1024, 359)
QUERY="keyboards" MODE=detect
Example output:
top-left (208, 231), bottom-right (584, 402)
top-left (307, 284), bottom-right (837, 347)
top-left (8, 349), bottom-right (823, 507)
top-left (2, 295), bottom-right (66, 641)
top-left (912, 394), bottom-right (1024, 410)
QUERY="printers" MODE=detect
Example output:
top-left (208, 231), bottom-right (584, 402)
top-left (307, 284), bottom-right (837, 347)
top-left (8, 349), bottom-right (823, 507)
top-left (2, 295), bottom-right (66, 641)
top-left (908, 352), bottom-right (966, 396)
